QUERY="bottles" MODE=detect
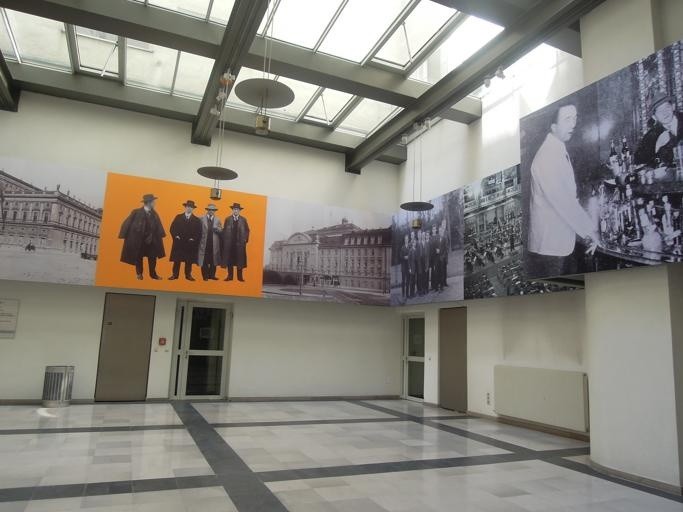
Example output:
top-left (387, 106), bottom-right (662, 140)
top-left (608, 136), bottom-right (632, 177)
top-left (597, 182), bottom-right (682, 260)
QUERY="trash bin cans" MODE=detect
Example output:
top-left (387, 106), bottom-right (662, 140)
top-left (42, 366), bottom-right (74, 408)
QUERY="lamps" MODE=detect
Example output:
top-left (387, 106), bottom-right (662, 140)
top-left (400, 95), bottom-right (435, 229)
top-left (198, 0), bottom-right (298, 201)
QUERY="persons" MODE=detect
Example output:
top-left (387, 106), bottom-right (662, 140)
top-left (224, 203), bottom-right (249, 281)
top-left (400, 217), bottom-right (451, 298)
top-left (119, 194), bottom-right (166, 280)
top-left (526, 100), bottom-right (603, 278)
top-left (633, 92), bottom-right (683, 168)
top-left (198, 204), bottom-right (223, 281)
top-left (168, 200), bottom-right (201, 280)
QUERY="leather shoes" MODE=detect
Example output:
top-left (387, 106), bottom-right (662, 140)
top-left (224, 277), bottom-right (233, 281)
top-left (208, 276), bottom-right (217, 280)
top-left (137, 274), bottom-right (142, 280)
top-left (186, 276), bottom-right (195, 280)
top-left (168, 275), bottom-right (178, 280)
top-left (237, 277), bottom-right (243, 281)
top-left (149, 273), bottom-right (159, 279)
top-left (203, 275), bottom-right (207, 281)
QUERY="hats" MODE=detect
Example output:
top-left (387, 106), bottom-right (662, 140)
top-left (140, 194), bottom-right (158, 202)
top-left (182, 200), bottom-right (198, 209)
top-left (205, 204), bottom-right (218, 211)
top-left (229, 203), bottom-right (243, 210)
top-left (647, 92), bottom-right (676, 117)
